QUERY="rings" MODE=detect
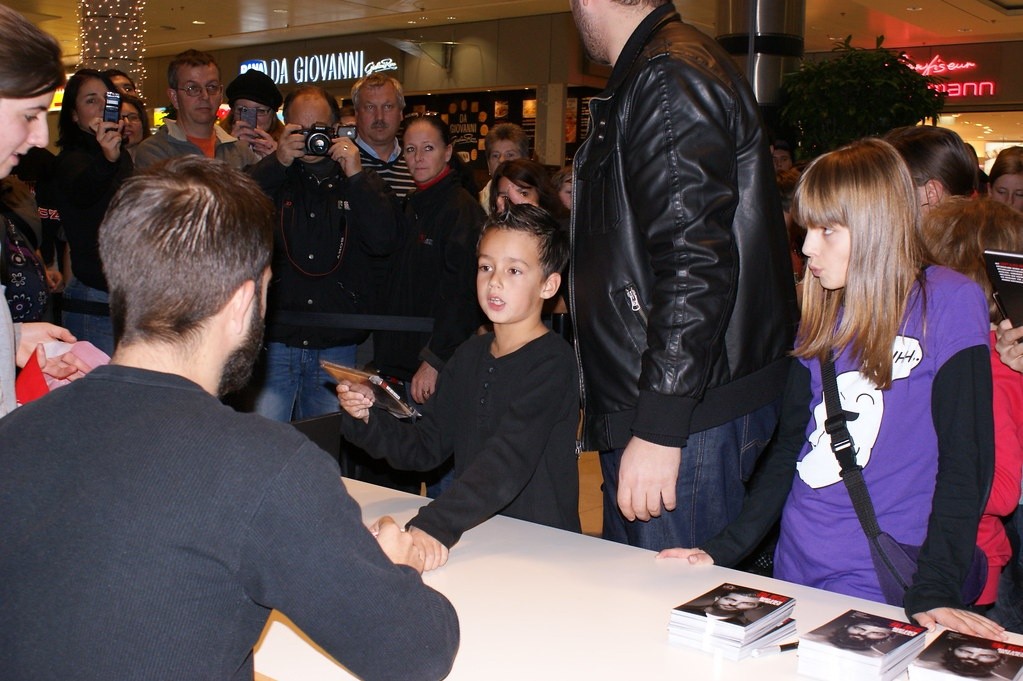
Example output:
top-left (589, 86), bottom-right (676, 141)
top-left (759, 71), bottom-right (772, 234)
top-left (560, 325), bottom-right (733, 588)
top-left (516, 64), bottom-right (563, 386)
top-left (344, 145), bottom-right (349, 151)
top-left (267, 144), bottom-right (274, 150)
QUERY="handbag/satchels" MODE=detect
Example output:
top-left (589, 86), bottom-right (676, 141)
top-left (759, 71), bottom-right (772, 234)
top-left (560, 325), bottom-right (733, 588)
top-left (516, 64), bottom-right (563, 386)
top-left (15, 340), bottom-right (112, 408)
top-left (2, 218), bottom-right (49, 324)
top-left (869, 532), bottom-right (988, 608)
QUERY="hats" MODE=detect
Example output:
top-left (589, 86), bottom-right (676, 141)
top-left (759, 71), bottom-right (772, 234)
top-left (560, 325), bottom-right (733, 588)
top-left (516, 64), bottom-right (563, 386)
top-left (226, 69), bottom-right (283, 112)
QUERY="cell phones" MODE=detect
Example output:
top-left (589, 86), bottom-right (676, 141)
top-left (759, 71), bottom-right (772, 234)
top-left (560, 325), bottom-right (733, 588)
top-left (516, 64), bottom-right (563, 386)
top-left (103, 92), bottom-right (120, 132)
top-left (240, 107), bottom-right (257, 139)
top-left (337, 126), bottom-right (357, 141)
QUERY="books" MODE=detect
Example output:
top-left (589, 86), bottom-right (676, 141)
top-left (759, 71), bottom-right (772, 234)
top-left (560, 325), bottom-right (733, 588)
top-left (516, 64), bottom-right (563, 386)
top-left (664, 582), bottom-right (1023, 681)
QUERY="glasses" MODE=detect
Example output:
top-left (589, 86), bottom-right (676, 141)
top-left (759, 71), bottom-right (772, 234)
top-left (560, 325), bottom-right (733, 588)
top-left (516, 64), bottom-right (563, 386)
top-left (120, 112), bottom-right (141, 124)
top-left (171, 81), bottom-right (224, 97)
top-left (232, 105), bottom-right (271, 116)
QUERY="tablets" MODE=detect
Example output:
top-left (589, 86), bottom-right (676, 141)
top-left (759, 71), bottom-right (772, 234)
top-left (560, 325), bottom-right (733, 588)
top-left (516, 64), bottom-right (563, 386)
top-left (319, 359), bottom-right (415, 417)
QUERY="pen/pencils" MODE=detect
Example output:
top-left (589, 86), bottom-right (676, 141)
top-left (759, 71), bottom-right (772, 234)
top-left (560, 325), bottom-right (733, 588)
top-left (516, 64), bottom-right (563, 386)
top-left (752, 640), bottom-right (799, 654)
top-left (377, 380), bottom-right (401, 400)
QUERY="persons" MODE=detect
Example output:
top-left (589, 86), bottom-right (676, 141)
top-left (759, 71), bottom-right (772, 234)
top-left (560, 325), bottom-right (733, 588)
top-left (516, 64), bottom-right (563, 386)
top-left (336, 194), bottom-right (581, 572)
top-left (799, 617), bottom-right (897, 659)
top-left (0, 0), bottom-right (460, 681)
top-left (674, 587), bottom-right (764, 627)
top-left (552, 0), bottom-right (1023, 642)
top-left (909, 639), bottom-right (1012, 681)
top-left (55, 48), bottom-right (491, 424)
top-left (477, 123), bottom-right (573, 309)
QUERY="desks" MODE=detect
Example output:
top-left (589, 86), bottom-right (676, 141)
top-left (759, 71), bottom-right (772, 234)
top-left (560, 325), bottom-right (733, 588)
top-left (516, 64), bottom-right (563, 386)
top-left (244, 476), bottom-right (1023, 681)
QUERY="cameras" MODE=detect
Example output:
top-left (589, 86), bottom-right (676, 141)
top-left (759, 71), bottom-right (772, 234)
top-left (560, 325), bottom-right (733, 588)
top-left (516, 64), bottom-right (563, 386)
top-left (291, 122), bottom-right (339, 157)
